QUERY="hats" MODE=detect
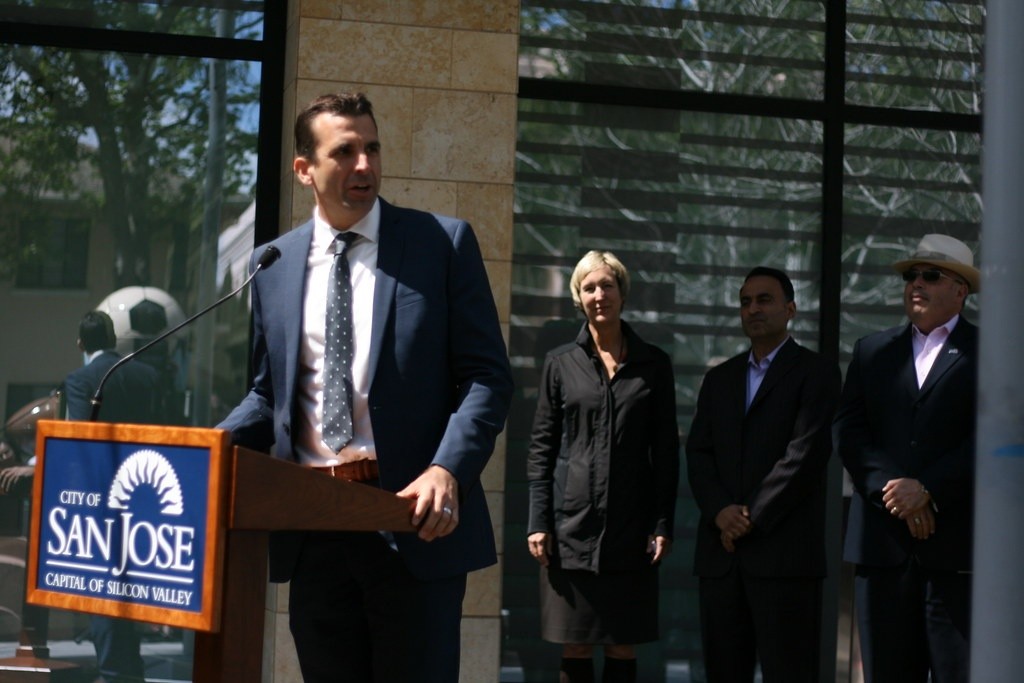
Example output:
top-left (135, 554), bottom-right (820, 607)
top-left (893, 234), bottom-right (981, 293)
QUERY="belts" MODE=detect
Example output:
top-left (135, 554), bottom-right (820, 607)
top-left (311, 459), bottom-right (379, 481)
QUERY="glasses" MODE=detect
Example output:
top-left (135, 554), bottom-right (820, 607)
top-left (903, 269), bottom-right (963, 285)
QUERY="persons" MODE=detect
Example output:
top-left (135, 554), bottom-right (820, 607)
top-left (0, 311), bottom-right (159, 683)
top-left (0, 436), bottom-right (21, 467)
top-left (683, 266), bottom-right (843, 683)
top-left (222, 90), bottom-right (514, 683)
top-left (526, 249), bottom-right (681, 683)
top-left (833, 232), bottom-right (981, 683)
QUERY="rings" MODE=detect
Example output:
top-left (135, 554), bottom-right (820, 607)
top-left (444, 507), bottom-right (451, 515)
top-left (915, 517), bottom-right (920, 524)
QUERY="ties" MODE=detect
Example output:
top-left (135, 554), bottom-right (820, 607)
top-left (321, 231), bottom-right (358, 456)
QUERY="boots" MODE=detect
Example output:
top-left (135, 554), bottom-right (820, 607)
top-left (602, 657), bottom-right (637, 683)
top-left (560, 657), bottom-right (594, 683)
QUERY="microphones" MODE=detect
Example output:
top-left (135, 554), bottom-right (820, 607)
top-left (88, 244), bottom-right (282, 420)
